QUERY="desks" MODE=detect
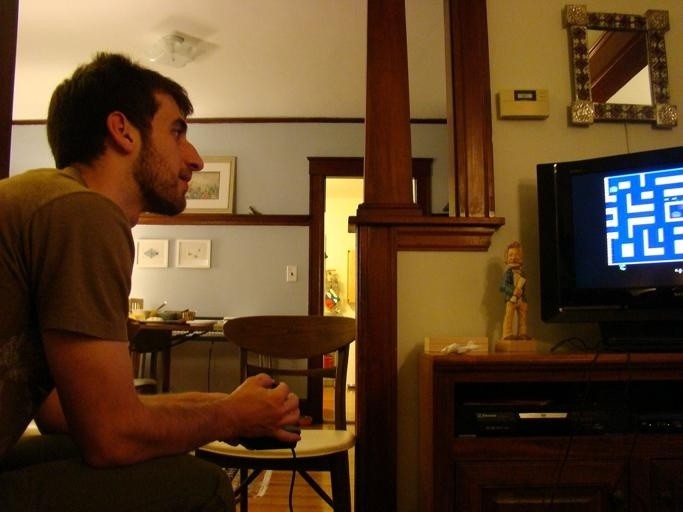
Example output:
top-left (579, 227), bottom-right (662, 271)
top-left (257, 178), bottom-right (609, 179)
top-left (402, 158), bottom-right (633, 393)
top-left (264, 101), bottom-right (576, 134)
top-left (128, 317), bottom-right (271, 382)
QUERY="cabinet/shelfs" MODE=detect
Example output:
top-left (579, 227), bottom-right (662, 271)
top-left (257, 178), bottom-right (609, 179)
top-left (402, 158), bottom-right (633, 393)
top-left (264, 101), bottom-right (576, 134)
top-left (418, 349), bottom-right (682, 512)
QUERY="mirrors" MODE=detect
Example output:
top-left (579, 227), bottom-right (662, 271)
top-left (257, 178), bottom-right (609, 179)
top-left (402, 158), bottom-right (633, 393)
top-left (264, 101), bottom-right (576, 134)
top-left (564, 2), bottom-right (677, 129)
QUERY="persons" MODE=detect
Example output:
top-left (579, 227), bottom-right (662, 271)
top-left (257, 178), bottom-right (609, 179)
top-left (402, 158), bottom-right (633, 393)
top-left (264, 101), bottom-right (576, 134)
top-left (495, 241), bottom-right (536, 352)
top-left (0, 51), bottom-right (301, 512)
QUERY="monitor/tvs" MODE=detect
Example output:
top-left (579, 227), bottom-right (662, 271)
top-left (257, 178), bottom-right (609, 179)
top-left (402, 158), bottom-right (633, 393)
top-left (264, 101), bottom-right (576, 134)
top-left (536, 144), bottom-right (683, 356)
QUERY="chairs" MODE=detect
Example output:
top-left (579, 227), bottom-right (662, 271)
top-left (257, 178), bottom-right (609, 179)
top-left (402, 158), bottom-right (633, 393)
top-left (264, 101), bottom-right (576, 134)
top-left (129, 328), bottom-right (171, 395)
top-left (193, 314), bottom-right (356, 512)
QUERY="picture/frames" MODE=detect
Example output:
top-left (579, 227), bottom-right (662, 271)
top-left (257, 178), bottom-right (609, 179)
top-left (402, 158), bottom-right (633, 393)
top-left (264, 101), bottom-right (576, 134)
top-left (134, 238), bottom-right (213, 271)
top-left (181, 155), bottom-right (238, 214)
